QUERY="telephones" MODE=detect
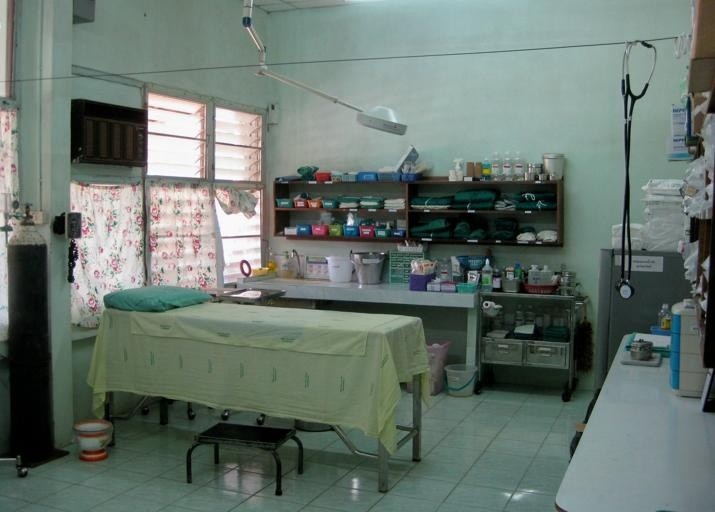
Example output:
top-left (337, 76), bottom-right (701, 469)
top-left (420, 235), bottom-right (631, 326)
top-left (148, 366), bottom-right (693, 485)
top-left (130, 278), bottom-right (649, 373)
top-left (68, 213), bottom-right (81, 238)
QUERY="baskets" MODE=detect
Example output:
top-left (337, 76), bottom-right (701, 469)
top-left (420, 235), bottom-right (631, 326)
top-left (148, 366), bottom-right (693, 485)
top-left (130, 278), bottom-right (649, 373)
top-left (524, 280), bottom-right (558, 295)
top-left (456, 255), bottom-right (494, 294)
top-left (276, 170), bottom-right (423, 236)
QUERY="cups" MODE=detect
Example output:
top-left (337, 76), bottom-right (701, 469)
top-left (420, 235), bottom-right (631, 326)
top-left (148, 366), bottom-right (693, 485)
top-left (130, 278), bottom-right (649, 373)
top-left (543, 154), bottom-right (568, 181)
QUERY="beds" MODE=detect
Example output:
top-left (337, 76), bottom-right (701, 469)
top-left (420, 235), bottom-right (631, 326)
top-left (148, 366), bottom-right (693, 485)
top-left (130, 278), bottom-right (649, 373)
top-left (102, 301), bottom-right (425, 492)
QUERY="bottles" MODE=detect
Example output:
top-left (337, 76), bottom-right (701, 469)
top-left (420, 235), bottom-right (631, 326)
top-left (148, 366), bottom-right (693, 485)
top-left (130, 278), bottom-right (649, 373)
top-left (449, 170), bottom-right (456, 181)
top-left (492, 303), bottom-right (568, 328)
top-left (481, 258), bottom-right (554, 293)
top-left (658, 304), bottom-right (672, 330)
top-left (560, 270), bottom-right (577, 295)
top-left (466, 150), bottom-right (556, 181)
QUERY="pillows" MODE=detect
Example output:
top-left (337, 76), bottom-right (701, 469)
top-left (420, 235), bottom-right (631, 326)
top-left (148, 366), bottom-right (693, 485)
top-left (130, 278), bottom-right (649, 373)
top-left (102, 284), bottom-right (214, 312)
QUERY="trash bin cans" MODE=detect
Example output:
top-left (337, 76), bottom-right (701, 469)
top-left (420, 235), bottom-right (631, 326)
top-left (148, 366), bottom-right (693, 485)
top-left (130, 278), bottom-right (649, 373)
top-left (407, 338), bottom-right (450, 395)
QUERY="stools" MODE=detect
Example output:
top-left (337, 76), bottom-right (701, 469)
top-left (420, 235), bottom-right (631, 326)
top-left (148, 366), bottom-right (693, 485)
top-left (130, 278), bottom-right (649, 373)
top-left (186, 422), bottom-right (304, 495)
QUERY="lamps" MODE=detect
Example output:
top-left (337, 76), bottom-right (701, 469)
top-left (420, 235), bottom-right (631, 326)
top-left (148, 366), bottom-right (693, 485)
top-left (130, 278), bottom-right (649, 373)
top-left (6, 202), bottom-right (44, 248)
top-left (240, 0), bottom-right (408, 135)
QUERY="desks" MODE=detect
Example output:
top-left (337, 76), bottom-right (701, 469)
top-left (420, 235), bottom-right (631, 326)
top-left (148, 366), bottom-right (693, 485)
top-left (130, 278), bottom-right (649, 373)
top-left (235, 276), bottom-right (479, 371)
top-left (554, 332), bottom-right (714, 511)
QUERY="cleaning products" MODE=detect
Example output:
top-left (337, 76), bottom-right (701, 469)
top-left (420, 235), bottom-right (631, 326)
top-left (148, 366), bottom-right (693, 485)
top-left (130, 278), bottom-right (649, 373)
top-left (453, 158), bottom-right (464, 181)
top-left (482, 158), bottom-right (491, 180)
top-left (481, 259), bottom-right (492, 292)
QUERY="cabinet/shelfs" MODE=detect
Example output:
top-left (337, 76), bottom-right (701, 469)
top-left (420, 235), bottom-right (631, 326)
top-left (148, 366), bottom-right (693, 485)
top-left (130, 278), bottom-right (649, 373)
top-left (71, 98), bottom-right (147, 168)
top-left (473, 290), bottom-right (590, 401)
top-left (272, 175), bottom-right (565, 248)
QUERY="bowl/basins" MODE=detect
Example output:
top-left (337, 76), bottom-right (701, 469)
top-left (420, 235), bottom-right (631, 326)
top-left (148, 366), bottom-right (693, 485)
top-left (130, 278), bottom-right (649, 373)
top-left (631, 339), bottom-right (653, 361)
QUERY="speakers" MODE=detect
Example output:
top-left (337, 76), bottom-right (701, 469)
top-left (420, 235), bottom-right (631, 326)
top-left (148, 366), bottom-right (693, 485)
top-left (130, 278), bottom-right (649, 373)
top-left (73, 0), bottom-right (95, 24)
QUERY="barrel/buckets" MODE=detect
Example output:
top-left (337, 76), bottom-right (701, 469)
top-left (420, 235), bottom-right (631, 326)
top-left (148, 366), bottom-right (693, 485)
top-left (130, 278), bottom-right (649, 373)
top-left (349, 249), bottom-right (389, 284)
top-left (325, 256), bottom-right (353, 283)
top-left (443, 364), bottom-right (478, 396)
